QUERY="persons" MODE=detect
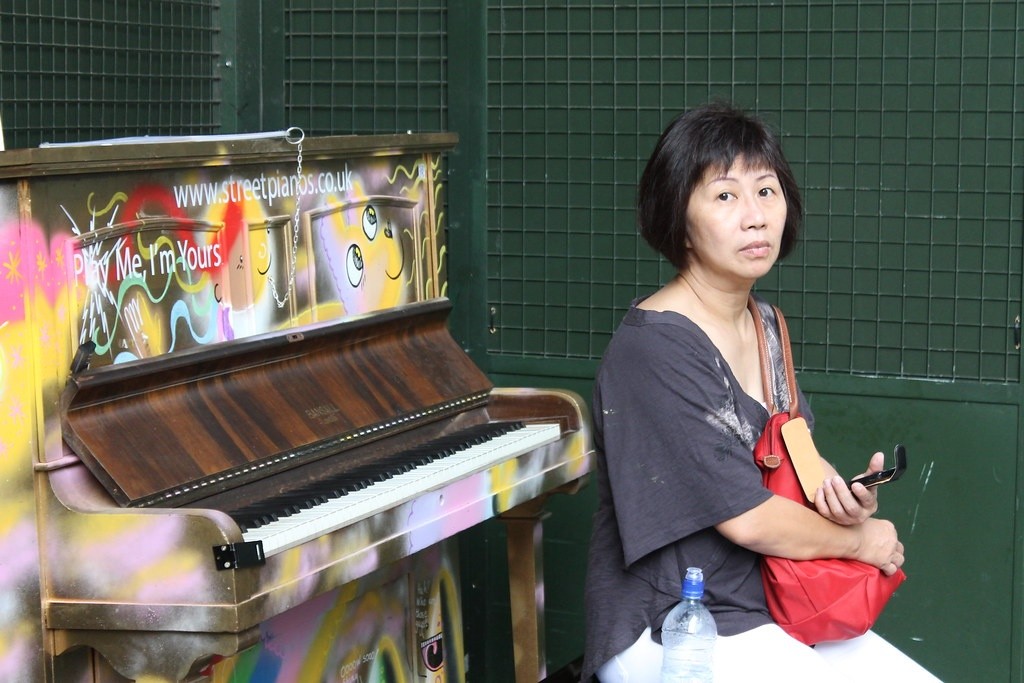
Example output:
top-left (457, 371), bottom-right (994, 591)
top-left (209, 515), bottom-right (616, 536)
top-left (577, 102), bottom-right (944, 683)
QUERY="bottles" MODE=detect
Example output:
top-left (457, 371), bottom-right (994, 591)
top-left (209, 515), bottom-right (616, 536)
top-left (657, 567), bottom-right (717, 682)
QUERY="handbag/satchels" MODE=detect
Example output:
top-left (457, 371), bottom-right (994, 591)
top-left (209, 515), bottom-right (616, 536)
top-left (749, 295), bottom-right (908, 649)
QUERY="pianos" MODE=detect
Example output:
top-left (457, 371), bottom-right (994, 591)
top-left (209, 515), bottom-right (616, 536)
top-left (1, 126), bottom-right (601, 682)
